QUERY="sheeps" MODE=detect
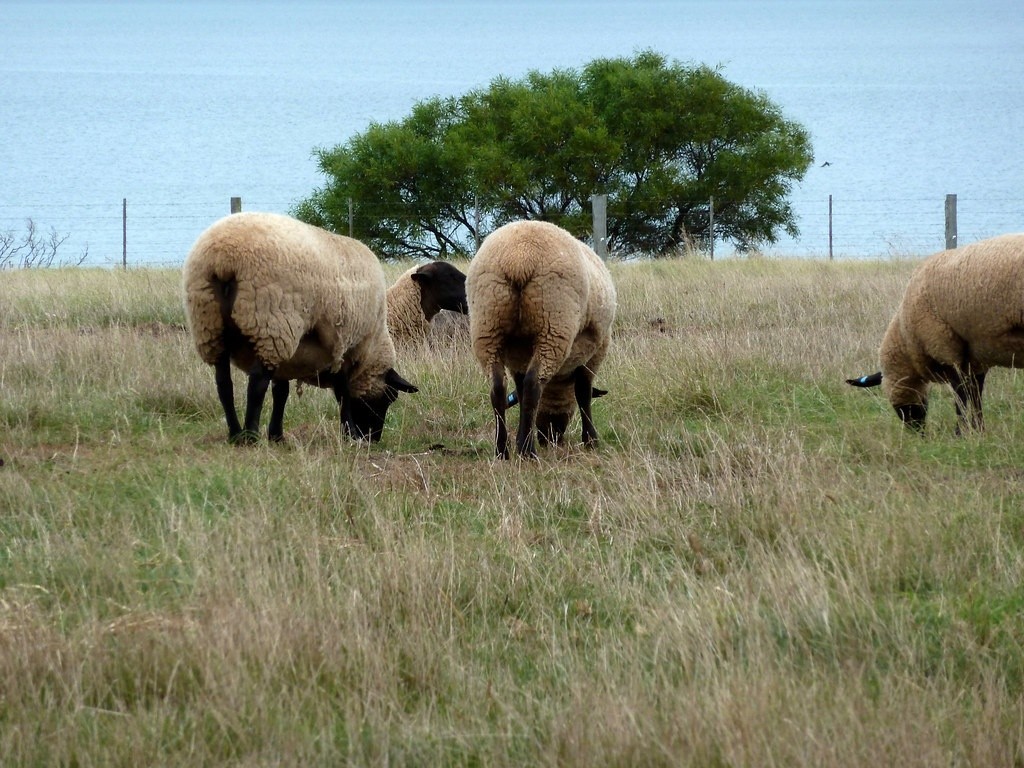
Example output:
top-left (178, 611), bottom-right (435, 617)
top-left (466, 219), bottom-right (617, 459)
top-left (845, 234), bottom-right (1024, 431)
top-left (183, 212), bottom-right (469, 446)
